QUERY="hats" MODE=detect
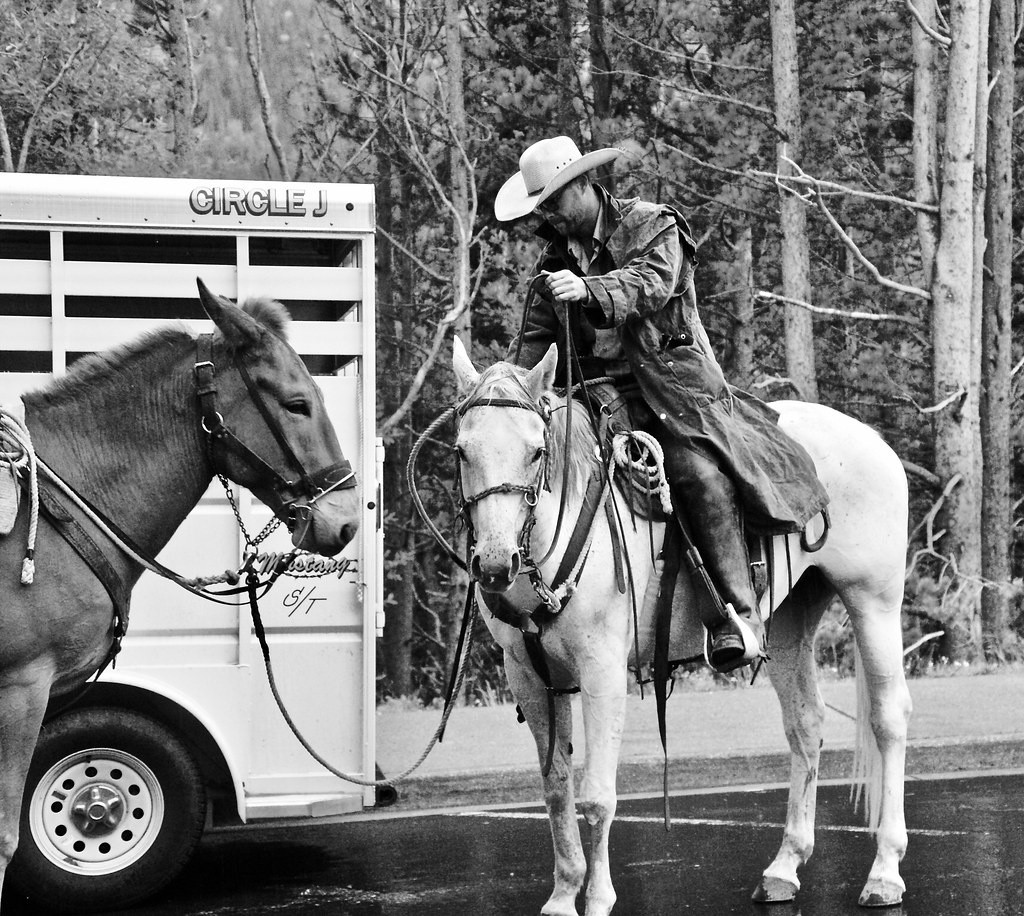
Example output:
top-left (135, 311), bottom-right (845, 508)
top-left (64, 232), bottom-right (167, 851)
top-left (493, 135), bottom-right (627, 222)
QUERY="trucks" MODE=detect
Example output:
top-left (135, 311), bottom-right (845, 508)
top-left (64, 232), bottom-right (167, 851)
top-left (2, 168), bottom-right (387, 903)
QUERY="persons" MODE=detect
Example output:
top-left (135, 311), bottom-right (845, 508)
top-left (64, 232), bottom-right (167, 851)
top-left (487, 136), bottom-right (831, 665)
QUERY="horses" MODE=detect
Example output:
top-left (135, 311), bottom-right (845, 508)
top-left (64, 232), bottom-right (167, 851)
top-left (450, 334), bottom-right (915, 916)
top-left (0, 276), bottom-right (362, 893)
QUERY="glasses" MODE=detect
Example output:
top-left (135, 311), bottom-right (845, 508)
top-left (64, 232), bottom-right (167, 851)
top-left (535, 185), bottom-right (566, 213)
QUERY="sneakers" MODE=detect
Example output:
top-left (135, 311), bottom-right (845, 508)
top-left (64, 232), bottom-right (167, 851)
top-left (711, 617), bottom-right (769, 665)
